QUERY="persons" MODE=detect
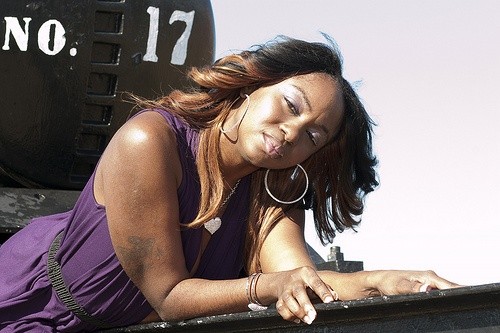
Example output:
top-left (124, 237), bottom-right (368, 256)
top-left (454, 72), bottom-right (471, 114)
top-left (0, 32), bottom-right (466, 333)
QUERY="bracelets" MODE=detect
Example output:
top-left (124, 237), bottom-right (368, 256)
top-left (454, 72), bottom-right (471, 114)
top-left (246, 272), bottom-right (268, 311)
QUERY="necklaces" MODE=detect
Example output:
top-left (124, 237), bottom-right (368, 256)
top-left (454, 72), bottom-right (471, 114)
top-left (204, 179), bottom-right (241, 234)
